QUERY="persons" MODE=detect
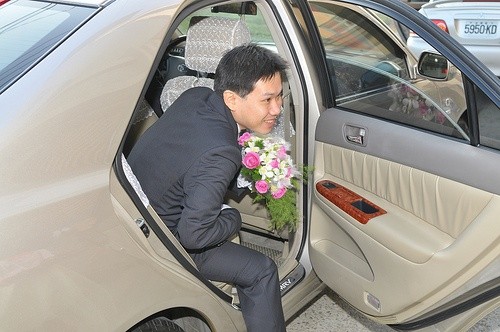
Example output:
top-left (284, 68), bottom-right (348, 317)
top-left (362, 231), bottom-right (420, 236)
top-left (126, 43), bottom-right (285, 332)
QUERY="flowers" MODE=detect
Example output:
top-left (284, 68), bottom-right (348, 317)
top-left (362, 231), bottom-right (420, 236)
top-left (238, 132), bottom-right (316, 233)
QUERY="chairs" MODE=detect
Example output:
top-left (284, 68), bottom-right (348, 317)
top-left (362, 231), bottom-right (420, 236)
top-left (160, 16), bottom-right (295, 240)
top-left (123, 95), bottom-right (160, 160)
